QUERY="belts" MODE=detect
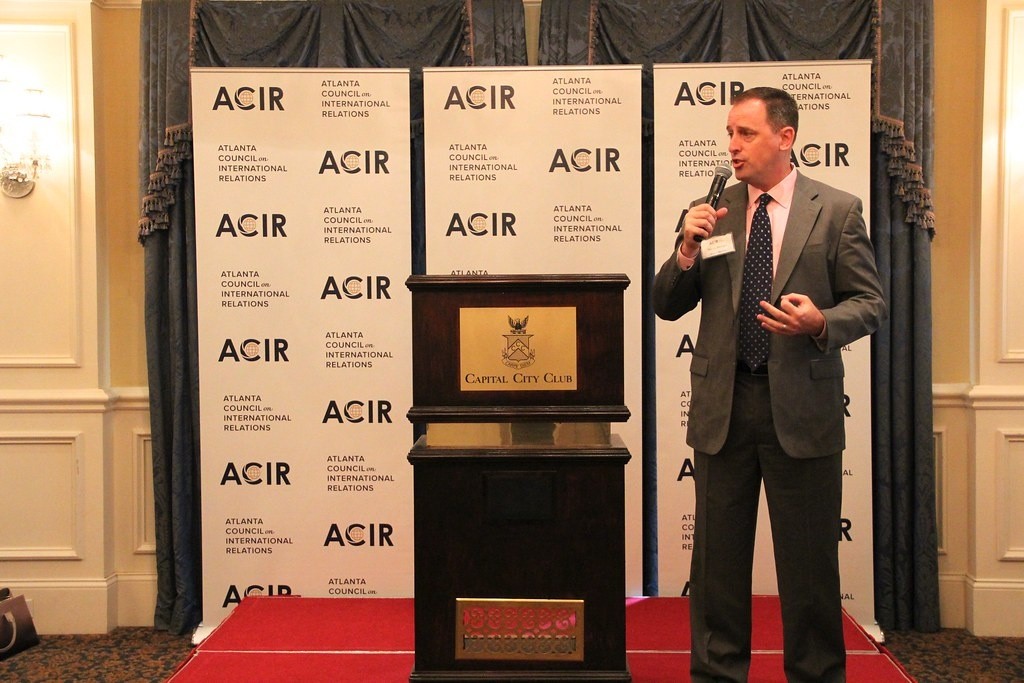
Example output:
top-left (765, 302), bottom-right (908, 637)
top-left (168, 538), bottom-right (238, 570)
top-left (736, 364), bottom-right (768, 374)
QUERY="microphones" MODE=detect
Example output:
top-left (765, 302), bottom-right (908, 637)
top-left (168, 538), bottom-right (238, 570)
top-left (693, 162), bottom-right (733, 242)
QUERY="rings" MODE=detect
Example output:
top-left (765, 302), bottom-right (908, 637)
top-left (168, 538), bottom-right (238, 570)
top-left (781, 324), bottom-right (786, 331)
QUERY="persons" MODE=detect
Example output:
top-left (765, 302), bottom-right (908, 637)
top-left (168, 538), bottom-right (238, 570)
top-left (652, 86), bottom-right (887, 683)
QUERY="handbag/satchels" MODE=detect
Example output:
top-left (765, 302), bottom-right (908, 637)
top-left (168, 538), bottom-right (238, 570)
top-left (0, 587), bottom-right (41, 661)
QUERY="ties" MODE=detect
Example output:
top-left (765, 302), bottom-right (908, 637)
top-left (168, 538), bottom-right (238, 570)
top-left (737, 193), bottom-right (774, 369)
